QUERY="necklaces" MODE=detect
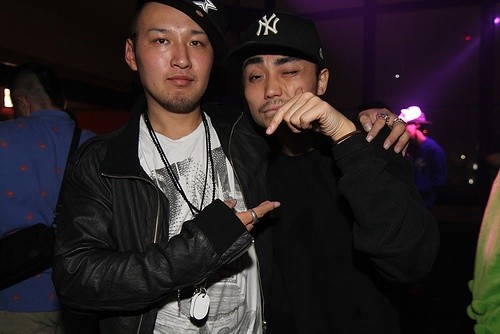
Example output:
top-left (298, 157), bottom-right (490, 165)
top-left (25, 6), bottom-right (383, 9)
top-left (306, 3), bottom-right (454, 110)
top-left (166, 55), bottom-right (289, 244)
top-left (144, 110), bottom-right (217, 320)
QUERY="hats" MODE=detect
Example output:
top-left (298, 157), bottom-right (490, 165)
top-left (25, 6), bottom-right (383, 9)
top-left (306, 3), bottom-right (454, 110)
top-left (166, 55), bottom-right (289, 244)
top-left (157, 0), bottom-right (230, 41)
top-left (227, 10), bottom-right (327, 86)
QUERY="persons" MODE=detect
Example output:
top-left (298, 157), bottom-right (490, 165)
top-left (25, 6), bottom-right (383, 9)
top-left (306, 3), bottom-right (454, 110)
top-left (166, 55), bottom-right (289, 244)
top-left (0, 61), bottom-right (99, 334)
top-left (223, 13), bottom-right (439, 334)
top-left (398, 105), bottom-right (446, 208)
top-left (52, 0), bottom-right (408, 334)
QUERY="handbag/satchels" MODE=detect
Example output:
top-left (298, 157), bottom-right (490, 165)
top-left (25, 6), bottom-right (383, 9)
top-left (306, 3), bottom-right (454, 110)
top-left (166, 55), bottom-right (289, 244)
top-left (0, 224), bottom-right (57, 291)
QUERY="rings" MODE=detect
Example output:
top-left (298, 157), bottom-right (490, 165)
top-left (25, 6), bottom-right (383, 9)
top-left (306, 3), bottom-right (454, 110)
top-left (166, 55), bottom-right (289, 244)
top-left (249, 209), bottom-right (259, 224)
top-left (377, 113), bottom-right (389, 123)
top-left (392, 119), bottom-right (407, 127)
